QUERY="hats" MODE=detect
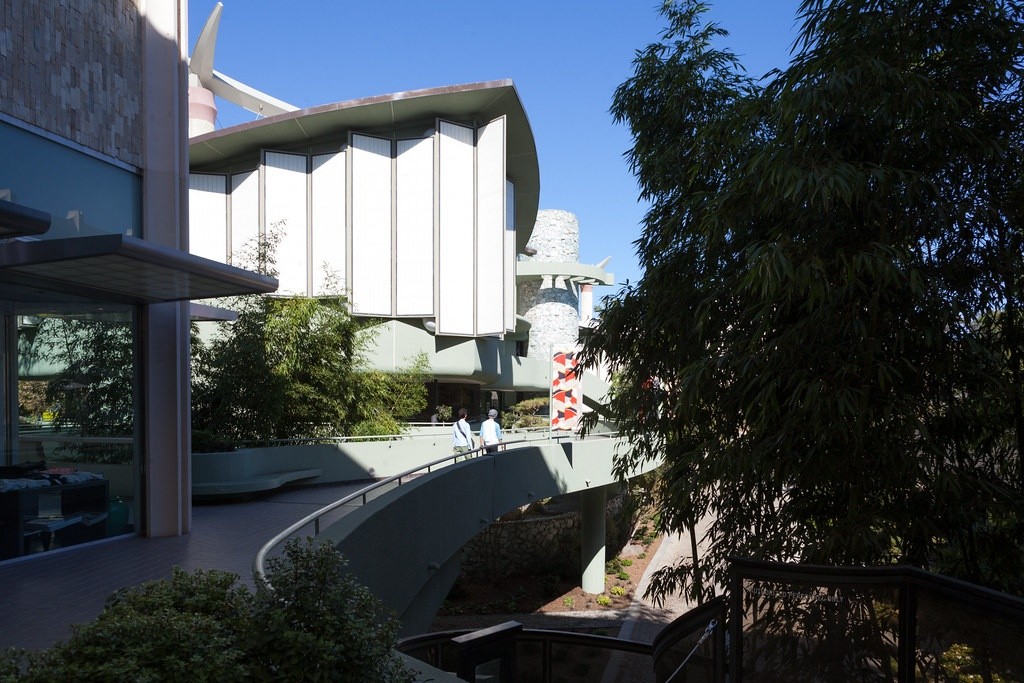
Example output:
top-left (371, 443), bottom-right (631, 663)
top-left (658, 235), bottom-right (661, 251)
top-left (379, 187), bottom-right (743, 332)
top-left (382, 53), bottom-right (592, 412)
top-left (488, 408), bottom-right (498, 416)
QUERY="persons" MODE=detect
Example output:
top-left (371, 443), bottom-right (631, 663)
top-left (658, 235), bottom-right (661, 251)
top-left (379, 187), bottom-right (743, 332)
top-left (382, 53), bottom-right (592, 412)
top-left (453, 409), bottom-right (473, 462)
top-left (480, 409), bottom-right (503, 455)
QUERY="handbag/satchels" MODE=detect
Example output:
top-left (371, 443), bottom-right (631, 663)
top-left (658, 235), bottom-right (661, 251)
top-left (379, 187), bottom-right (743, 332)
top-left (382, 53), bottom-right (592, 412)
top-left (468, 438), bottom-right (475, 451)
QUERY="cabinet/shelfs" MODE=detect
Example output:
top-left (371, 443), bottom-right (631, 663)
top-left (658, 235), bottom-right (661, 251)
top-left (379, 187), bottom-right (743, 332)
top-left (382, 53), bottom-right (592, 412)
top-left (0, 479), bottom-right (111, 559)
